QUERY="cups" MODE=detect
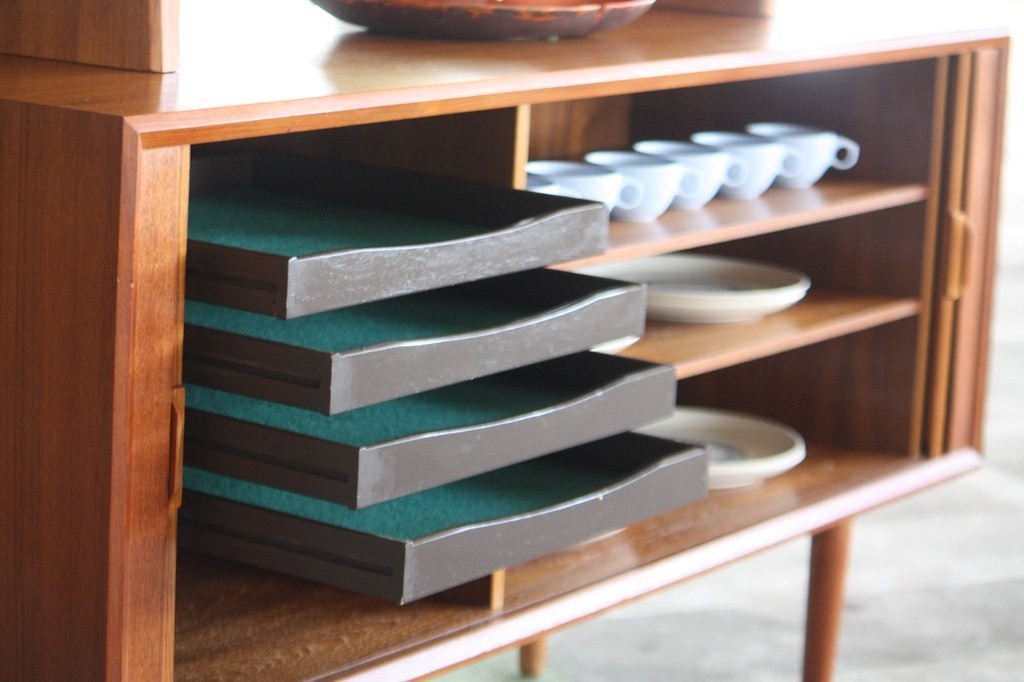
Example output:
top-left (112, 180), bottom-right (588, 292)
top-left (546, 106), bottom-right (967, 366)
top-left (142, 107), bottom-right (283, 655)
top-left (584, 150), bottom-right (699, 223)
top-left (745, 121), bottom-right (860, 188)
top-left (526, 159), bottom-right (644, 219)
top-left (633, 139), bottom-right (749, 211)
top-left (689, 130), bottom-right (805, 201)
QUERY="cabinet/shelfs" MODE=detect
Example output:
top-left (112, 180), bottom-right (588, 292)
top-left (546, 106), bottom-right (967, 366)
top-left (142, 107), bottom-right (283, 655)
top-left (0, 0), bottom-right (1009, 682)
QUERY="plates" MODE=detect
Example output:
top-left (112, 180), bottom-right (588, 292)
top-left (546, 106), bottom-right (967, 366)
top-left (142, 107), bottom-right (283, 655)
top-left (310, 0), bottom-right (658, 42)
top-left (633, 403), bottom-right (807, 489)
top-left (580, 253), bottom-right (812, 326)
